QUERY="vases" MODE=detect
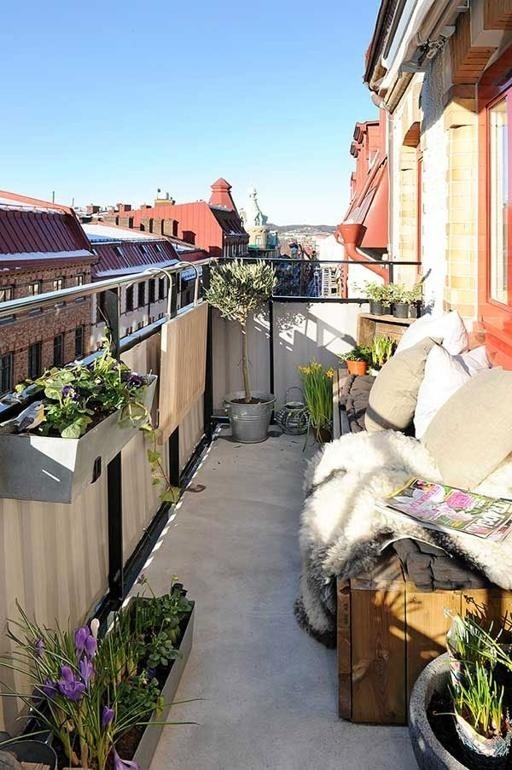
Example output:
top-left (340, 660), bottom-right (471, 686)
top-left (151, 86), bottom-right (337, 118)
top-left (313, 423), bottom-right (331, 445)
top-left (2, 371), bottom-right (158, 506)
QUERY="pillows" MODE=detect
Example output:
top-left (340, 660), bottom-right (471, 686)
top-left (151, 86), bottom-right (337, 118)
top-left (364, 306), bottom-right (512, 495)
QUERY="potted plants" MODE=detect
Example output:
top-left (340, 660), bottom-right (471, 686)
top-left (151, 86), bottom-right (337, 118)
top-left (71, 593), bottom-right (196, 770)
top-left (443, 662), bottom-right (512, 757)
top-left (340, 345), bottom-right (371, 377)
top-left (361, 281), bottom-right (424, 319)
top-left (200, 258), bottom-right (286, 447)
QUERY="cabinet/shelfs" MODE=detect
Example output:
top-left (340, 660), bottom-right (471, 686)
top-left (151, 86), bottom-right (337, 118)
top-left (355, 313), bottom-right (415, 352)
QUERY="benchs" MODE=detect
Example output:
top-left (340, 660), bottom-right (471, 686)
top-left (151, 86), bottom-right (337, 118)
top-left (330, 362), bottom-right (512, 728)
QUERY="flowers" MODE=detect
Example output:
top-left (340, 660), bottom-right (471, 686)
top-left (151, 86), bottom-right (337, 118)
top-left (442, 600), bottom-right (512, 696)
top-left (0, 597), bottom-right (144, 769)
top-left (295, 359), bottom-right (336, 452)
top-left (1, 345), bottom-right (202, 512)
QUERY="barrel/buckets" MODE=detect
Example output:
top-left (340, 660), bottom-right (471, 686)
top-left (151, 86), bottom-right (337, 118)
top-left (224, 390), bottom-right (277, 444)
